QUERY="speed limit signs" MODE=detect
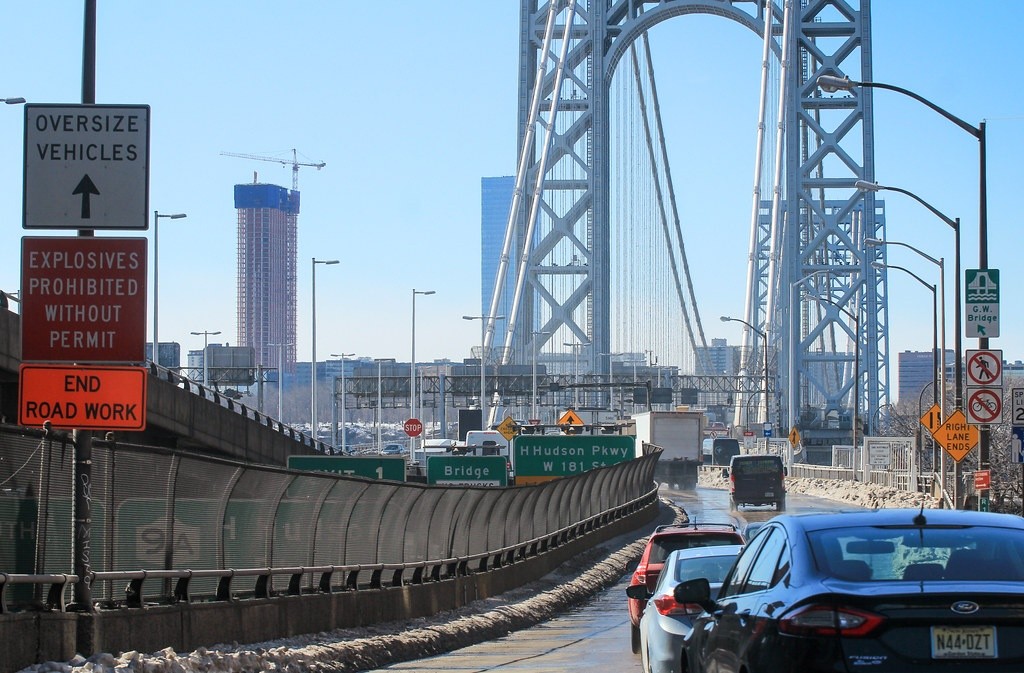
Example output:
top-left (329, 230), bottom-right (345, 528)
top-left (1010, 387), bottom-right (1024, 428)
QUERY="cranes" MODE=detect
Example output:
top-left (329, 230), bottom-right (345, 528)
top-left (220, 147), bottom-right (327, 190)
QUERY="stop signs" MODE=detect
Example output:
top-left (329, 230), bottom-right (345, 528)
top-left (404, 418), bottom-right (423, 438)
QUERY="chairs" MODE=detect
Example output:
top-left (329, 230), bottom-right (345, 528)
top-left (840, 549), bottom-right (1015, 580)
top-left (689, 563), bottom-right (720, 584)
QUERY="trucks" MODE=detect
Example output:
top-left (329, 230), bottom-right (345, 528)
top-left (630, 410), bottom-right (704, 491)
top-left (559, 410), bottom-right (621, 437)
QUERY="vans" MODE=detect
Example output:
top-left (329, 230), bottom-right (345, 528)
top-left (466, 430), bottom-right (515, 485)
top-left (713, 438), bottom-right (741, 477)
top-left (722, 454), bottom-right (788, 512)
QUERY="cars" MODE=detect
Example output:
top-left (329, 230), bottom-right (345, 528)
top-left (673, 511), bottom-right (1024, 673)
top-left (337, 445), bottom-right (356, 455)
top-left (703, 438), bottom-right (714, 454)
top-left (380, 444), bottom-right (404, 455)
top-left (625, 544), bottom-right (745, 673)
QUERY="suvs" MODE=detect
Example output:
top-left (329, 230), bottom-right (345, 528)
top-left (627, 521), bottom-right (746, 656)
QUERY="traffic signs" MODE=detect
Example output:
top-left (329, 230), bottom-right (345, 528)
top-left (20, 102), bottom-right (152, 229)
top-left (973, 469), bottom-right (991, 490)
top-left (964, 267), bottom-right (1001, 338)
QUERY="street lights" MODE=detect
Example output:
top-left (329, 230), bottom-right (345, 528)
top-left (814, 73), bottom-right (990, 512)
top-left (529, 330), bottom-right (550, 420)
top-left (855, 178), bottom-right (965, 511)
top-left (462, 314), bottom-right (505, 431)
top-left (331, 353), bottom-right (356, 454)
top-left (190, 330), bottom-right (222, 400)
top-left (868, 260), bottom-right (938, 499)
top-left (804, 294), bottom-right (860, 483)
top-left (863, 237), bottom-right (946, 503)
top-left (409, 289), bottom-right (437, 463)
top-left (720, 315), bottom-right (769, 455)
top-left (311, 258), bottom-right (339, 450)
top-left (623, 359), bottom-right (647, 390)
top-left (266, 342), bottom-right (294, 423)
top-left (918, 377), bottom-right (949, 484)
top-left (598, 352), bottom-right (623, 412)
top-left (564, 341), bottom-right (591, 412)
top-left (873, 403), bottom-right (893, 437)
top-left (152, 211), bottom-right (187, 370)
top-left (373, 358), bottom-right (396, 455)
